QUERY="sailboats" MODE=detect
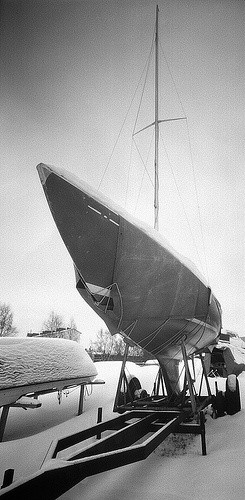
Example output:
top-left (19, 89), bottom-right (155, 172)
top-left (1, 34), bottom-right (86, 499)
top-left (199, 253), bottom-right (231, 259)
top-left (35, 5), bottom-right (221, 395)
